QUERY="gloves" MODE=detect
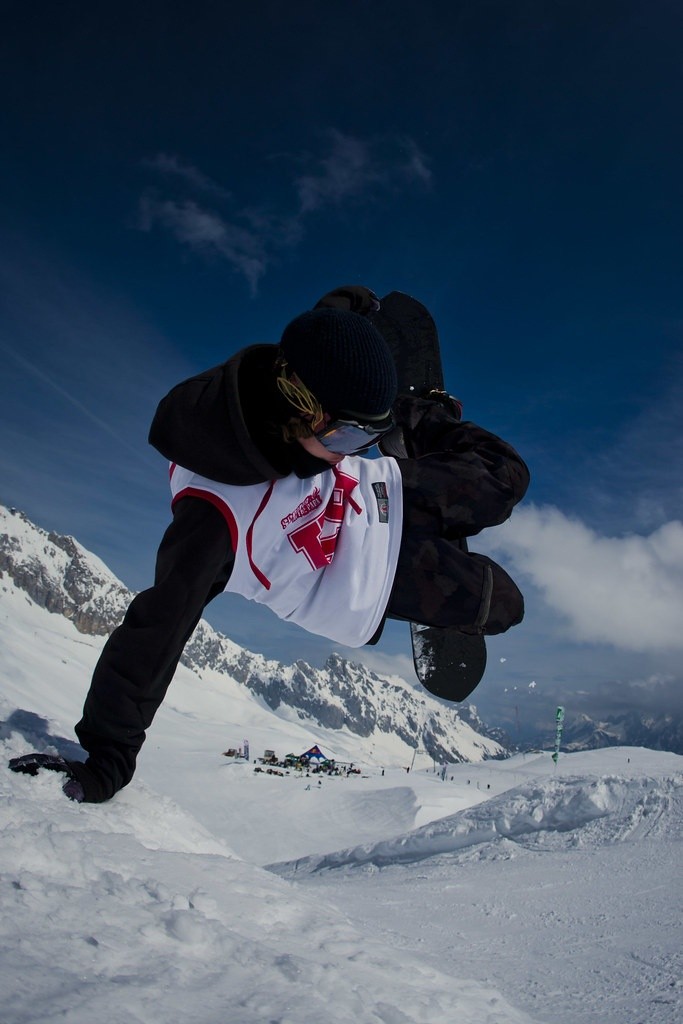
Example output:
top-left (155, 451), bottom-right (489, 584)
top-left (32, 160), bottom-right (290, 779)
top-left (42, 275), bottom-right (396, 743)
top-left (9, 745), bottom-right (84, 803)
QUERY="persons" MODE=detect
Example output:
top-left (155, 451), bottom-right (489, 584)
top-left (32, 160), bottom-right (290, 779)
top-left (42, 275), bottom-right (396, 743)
top-left (382, 769), bottom-right (384, 776)
top-left (6, 284), bottom-right (531, 801)
top-left (407, 767), bottom-right (409, 773)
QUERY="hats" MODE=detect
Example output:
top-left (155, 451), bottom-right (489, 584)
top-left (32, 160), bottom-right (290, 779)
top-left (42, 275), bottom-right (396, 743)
top-left (281, 307), bottom-right (401, 423)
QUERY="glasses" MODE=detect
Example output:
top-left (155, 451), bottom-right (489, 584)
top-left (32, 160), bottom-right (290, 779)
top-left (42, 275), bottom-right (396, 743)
top-left (313, 411), bottom-right (398, 457)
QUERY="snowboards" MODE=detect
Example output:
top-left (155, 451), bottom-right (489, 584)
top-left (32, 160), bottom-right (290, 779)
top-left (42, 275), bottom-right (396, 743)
top-left (379, 287), bottom-right (488, 704)
